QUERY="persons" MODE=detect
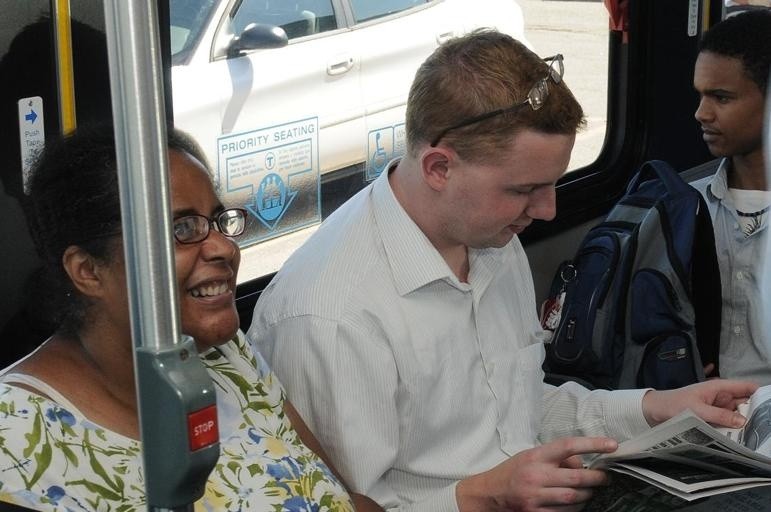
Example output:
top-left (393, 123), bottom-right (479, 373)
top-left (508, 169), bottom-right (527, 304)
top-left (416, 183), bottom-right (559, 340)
top-left (0, 126), bottom-right (385, 511)
top-left (247, 26), bottom-right (762, 510)
top-left (685, 12), bottom-right (771, 390)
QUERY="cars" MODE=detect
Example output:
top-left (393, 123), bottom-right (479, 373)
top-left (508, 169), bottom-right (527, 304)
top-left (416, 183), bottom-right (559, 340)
top-left (163, 1), bottom-right (527, 284)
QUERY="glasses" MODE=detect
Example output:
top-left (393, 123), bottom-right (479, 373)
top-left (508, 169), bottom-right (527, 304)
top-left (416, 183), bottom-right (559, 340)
top-left (430, 53), bottom-right (564, 148)
top-left (95, 208), bottom-right (248, 245)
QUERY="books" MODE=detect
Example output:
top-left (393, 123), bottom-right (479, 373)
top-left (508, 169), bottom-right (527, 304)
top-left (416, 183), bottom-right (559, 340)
top-left (586, 385), bottom-right (771, 511)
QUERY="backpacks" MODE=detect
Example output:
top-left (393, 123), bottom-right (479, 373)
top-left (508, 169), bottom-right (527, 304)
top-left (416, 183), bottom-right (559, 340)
top-left (539, 158), bottom-right (724, 393)
